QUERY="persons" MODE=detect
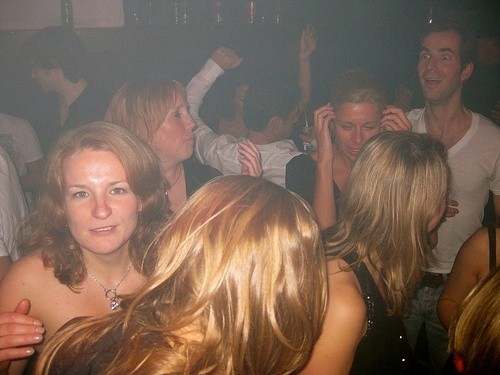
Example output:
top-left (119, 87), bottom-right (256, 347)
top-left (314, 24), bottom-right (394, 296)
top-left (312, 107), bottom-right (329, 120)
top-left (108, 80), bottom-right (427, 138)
top-left (447, 255), bottom-right (499, 375)
top-left (1, 149), bottom-right (33, 282)
top-left (1, 1), bottom-right (500, 194)
top-left (26, 174), bottom-right (328, 375)
top-left (285, 68), bottom-right (412, 241)
top-left (293, 130), bottom-right (451, 375)
top-left (1, 119), bottom-right (174, 374)
top-left (438, 215), bottom-right (499, 332)
top-left (392, 20), bottom-right (500, 375)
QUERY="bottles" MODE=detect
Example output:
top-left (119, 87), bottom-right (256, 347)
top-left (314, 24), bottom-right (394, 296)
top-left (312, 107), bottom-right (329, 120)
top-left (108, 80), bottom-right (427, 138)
top-left (302, 109), bottom-right (314, 152)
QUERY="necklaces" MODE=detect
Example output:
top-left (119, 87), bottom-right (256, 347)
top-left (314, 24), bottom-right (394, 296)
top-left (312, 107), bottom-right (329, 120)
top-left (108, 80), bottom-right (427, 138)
top-left (81, 257), bottom-right (138, 311)
